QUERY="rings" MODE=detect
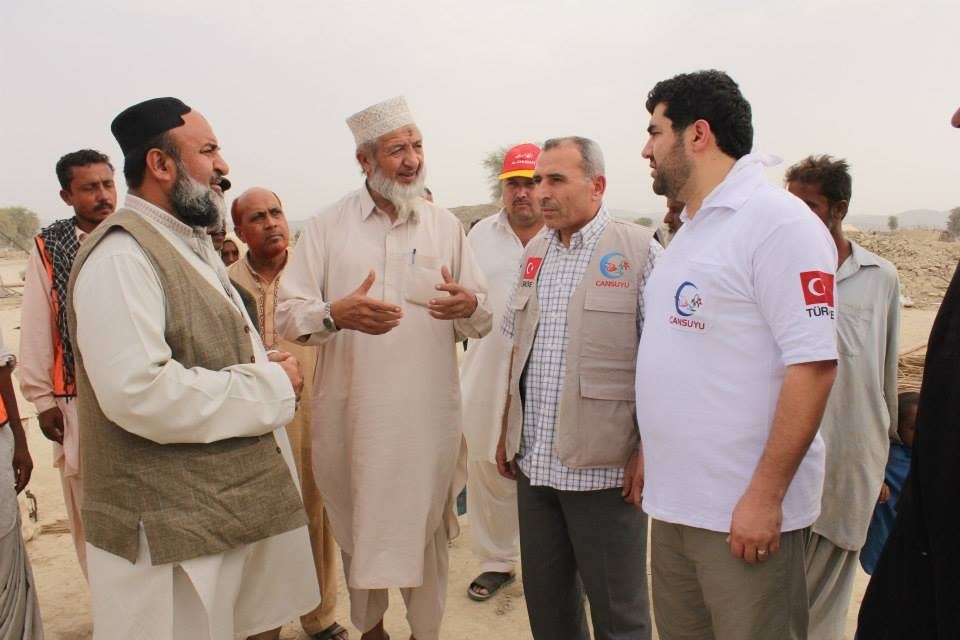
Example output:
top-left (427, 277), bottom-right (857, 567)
top-left (757, 549), bottom-right (768, 555)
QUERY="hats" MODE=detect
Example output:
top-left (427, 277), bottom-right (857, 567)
top-left (500, 143), bottom-right (541, 180)
top-left (111, 97), bottom-right (190, 155)
top-left (345, 95), bottom-right (414, 144)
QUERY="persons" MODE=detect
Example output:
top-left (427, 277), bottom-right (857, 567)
top-left (222, 187), bottom-right (349, 640)
top-left (633, 70), bottom-right (838, 640)
top-left (858, 391), bottom-right (929, 577)
top-left (853, 102), bottom-right (960, 640)
top-left (494, 136), bottom-right (655, 640)
top-left (783, 154), bottom-right (900, 639)
top-left (204, 217), bottom-right (242, 268)
top-left (663, 197), bottom-right (686, 235)
top-left (273, 95), bottom-right (494, 640)
top-left (0, 331), bottom-right (45, 639)
top-left (457, 144), bottom-right (549, 602)
top-left (67, 97), bottom-right (304, 640)
top-left (20, 150), bottom-right (117, 584)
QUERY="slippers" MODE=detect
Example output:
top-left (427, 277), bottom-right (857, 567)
top-left (311, 622), bottom-right (349, 640)
top-left (467, 571), bottom-right (514, 600)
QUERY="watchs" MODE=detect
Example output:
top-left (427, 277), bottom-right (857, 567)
top-left (321, 302), bottom-right (340, 334)
